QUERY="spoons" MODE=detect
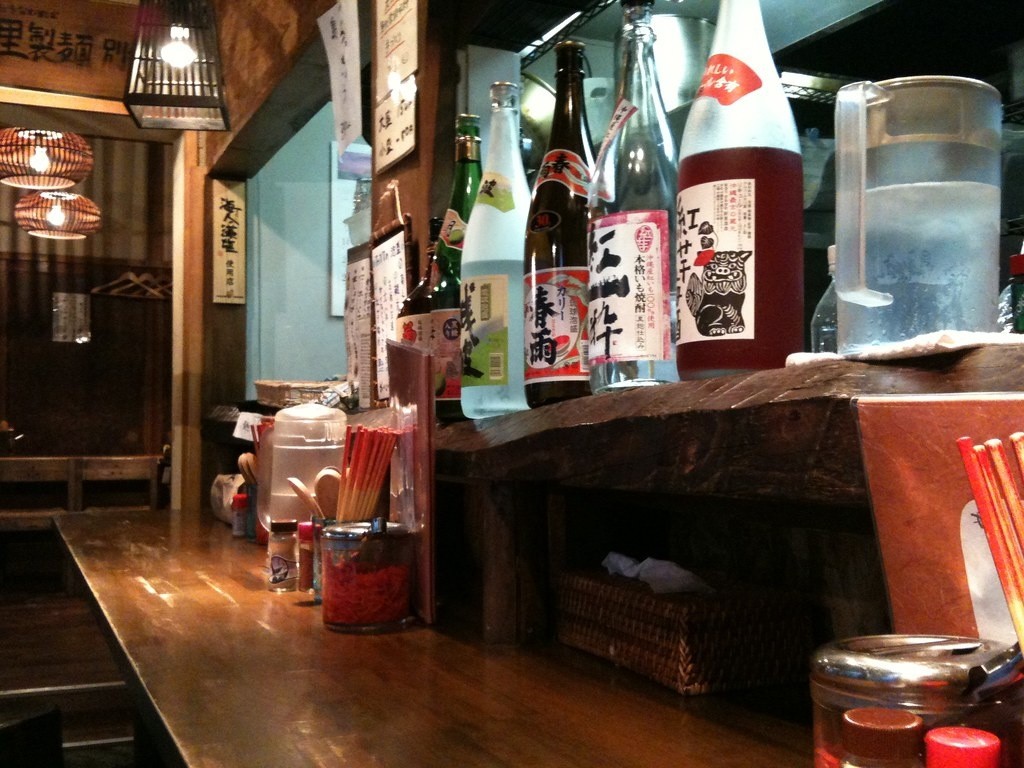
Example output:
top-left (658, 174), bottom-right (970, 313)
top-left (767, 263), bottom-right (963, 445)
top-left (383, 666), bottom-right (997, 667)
top-left (286, 466), bottom-right (342, 521)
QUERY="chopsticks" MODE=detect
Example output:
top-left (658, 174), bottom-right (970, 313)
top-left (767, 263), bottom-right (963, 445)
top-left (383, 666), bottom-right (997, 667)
top-left (250, 416), bottom-right (275, 458)
top-left (956, 432), bottom-right (1024, 661)
top-left (336, 423), bottom-right (400, 522)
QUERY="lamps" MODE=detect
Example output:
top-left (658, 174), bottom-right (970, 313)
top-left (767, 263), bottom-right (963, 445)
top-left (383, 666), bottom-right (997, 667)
top-left (121, 1), bottom-right (230, 132)
top-left (0, 125), bottom-right (96, 190)
top-left (14, 190), bottom-right (103, 240)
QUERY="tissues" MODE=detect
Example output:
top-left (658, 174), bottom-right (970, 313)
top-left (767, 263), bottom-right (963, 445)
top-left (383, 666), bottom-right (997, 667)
top-left (554, 551), bottom-right (793, 697)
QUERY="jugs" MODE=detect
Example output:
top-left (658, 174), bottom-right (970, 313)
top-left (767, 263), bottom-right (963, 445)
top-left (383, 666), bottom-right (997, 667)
top-left (258, 402), bottom-right (347, 532)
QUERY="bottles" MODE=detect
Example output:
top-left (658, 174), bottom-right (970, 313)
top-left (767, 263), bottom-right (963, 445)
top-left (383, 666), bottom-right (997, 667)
top-left (523, 41), bottom-right (595, 408)
top-left (838, 707), bottom-right (925, 768)
top-left (998, 254), bottom-right (1024, 335)
top-left (459, 81), bottom-right (529, 420)
top-left (298, 522), bottom-right (313, 592)
top-left (811, 245), bottom-right (838, 354)
top-left (925, 726), bottom-right (1002, 768)
top-left (398, 218), bottom-right (444, 357)
top-left (585, 1), bottom-right (679, 394)
top-left (676, 0), bottom-right (805, 380)
top-left (232, 492), bottom-right (248, 538)
top-left (427, 114), bottom-right (482, 427)
top-left (267, 519), bottom-right (298, 592)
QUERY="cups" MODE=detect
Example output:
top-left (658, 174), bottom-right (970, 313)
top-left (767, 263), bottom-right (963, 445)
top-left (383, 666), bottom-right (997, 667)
top-left (833, 75), bottom-right (1001, 354)
